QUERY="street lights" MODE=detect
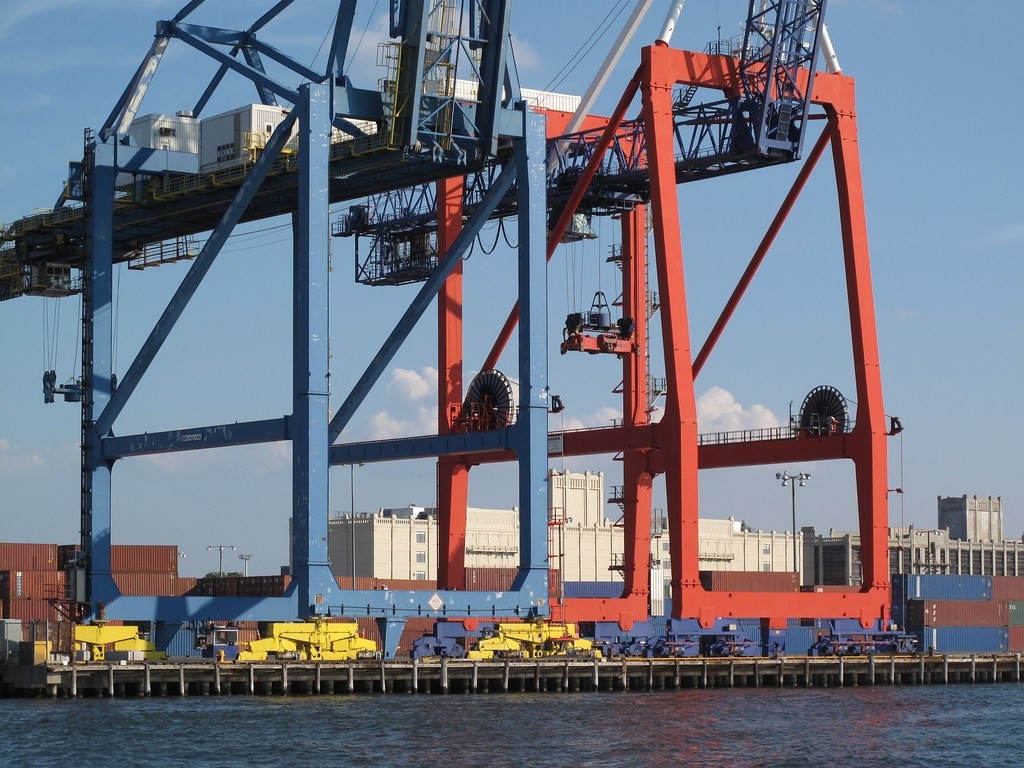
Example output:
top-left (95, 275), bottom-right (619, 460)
top-left (775, 470), bottom-right (811, 572)
top-left (207, 545), bottom-right (236, 578)
top-left (238, 553), bottom-right (253, 577)
top-left (344, 462), bottom-right (365, 589)
